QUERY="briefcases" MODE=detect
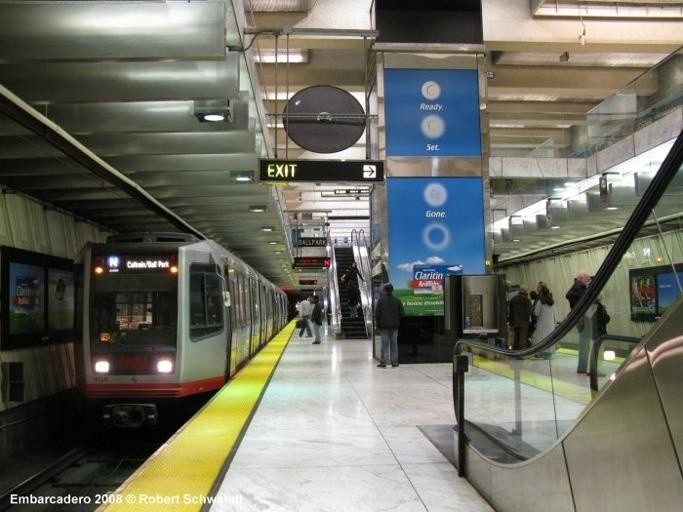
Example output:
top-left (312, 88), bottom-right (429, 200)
top-left (296, 320), bottom-right (308, 328)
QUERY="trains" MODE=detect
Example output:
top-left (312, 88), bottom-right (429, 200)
top-left (73, 231), bottom-right (289, 430)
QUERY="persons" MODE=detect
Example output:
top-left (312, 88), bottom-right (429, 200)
top-left (341, 261), bottom-right (366, 318)
top-left (565, 271), bottom-right (611, 377)
top-left (509, 281), bottom-right (556, 360)
top-left (296, 295), bottom-right (322, 344)
top-left (636, 278), bottom-right (656, 307)
top-left (375, 283), bottom-right (404, 368)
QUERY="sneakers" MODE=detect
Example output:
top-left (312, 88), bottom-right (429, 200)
top-left (299, 333), bottom-right (321, 344)
top-left (377, 363), bottom-right (399, 368)
top-left (577, 365), bottom-right (607, 377)
